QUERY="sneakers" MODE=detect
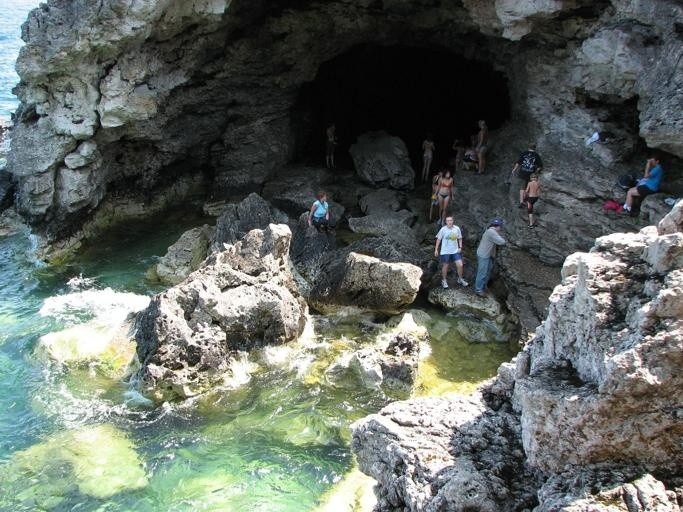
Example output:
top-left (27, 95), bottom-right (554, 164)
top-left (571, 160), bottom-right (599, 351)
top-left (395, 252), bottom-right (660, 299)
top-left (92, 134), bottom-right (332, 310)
top-left (528, 225), bottom-right (533, 229)
top-left (441, 280), bottom-right (448, 288)
top-left (620, 207), bottom-right (632, 215)
top-left (476, 287), bottom-right (489, 297)
top-left (519, 199), bottom-right (525, 207)
top-left (458, 278), bottom-right (468, 286)
top-left (437, 219), bottom-right (445, 226)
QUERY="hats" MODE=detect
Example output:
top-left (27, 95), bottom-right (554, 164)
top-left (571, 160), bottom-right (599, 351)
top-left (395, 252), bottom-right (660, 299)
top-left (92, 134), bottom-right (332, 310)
top-left (491, 218), bottom-right (502, 225)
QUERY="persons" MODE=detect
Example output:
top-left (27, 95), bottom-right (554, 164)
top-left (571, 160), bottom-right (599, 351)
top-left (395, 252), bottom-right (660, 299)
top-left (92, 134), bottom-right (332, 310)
top-left (421, 120), bottom-right (489, 227)
top-left (511, 143), bottom-right (542, 209)
top-left (523, 173), bottom-right (541, 229)
top-left (474, 217), bottom-right (505, 297)
top-left (325, 123), bottom-right (338, 168)
top-left (308, 191), bottom-right (336, 233)
top-left (434, 215), bottom-right (469, 288)
top-left (619, 153), bottom-right (665, 216)
